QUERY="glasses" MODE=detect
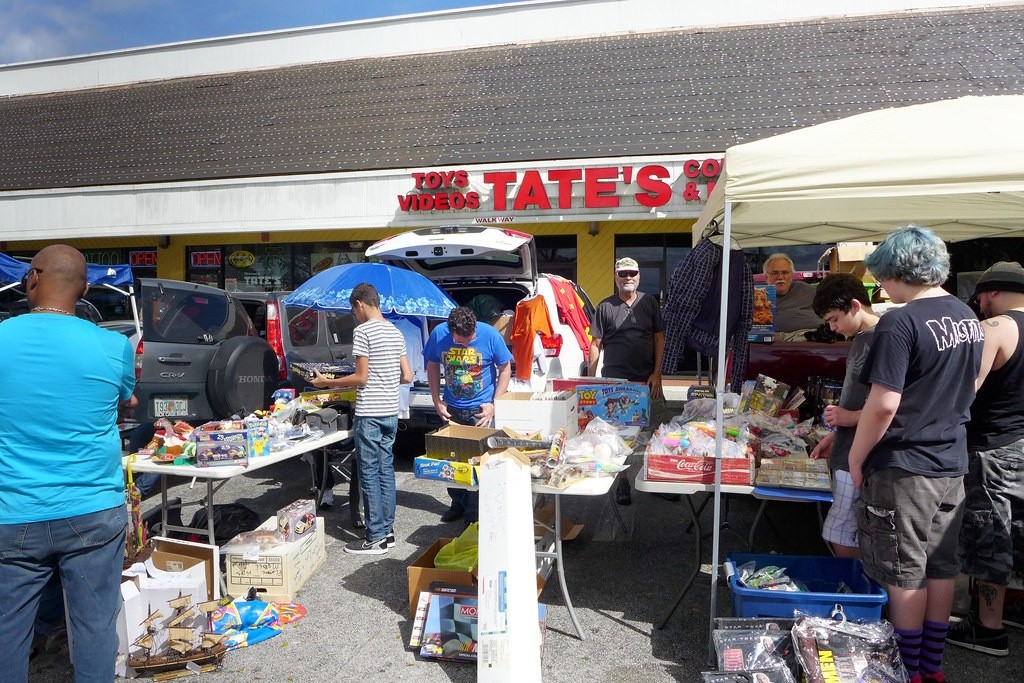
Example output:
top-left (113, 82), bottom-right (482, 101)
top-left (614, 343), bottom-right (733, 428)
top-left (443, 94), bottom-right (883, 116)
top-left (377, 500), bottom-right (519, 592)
top-left (21, 268), bottom-right (43, 286)
top-left (766, 269), bottom-right (794, 276)
top-left (453, 333), bottom-right (476, 344)
top-left (350, 302), bottom-right (357, 316)
top-left (616, 271), bottom-right (638, 278)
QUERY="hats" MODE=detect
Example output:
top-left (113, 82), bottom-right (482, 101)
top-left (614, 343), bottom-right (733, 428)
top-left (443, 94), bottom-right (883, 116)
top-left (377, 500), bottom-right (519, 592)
top-left (973, 260), bottom-right (1023, 293)
top-left (615, 256), bottom-right (639, 271)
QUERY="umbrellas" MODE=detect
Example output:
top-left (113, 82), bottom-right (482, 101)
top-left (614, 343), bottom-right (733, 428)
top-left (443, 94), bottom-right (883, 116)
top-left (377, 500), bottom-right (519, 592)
top-left (281, 263), bottom-right (460, 318)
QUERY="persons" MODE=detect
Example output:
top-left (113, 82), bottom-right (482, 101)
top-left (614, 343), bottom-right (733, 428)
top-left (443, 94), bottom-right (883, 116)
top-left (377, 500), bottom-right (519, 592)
top-left (310, 283), bottom-right (414, 555)
top-left (0, 244), bottom-right (138, 683)
top-left (810, 273), bottom-right (881, 556)
top-left (849, 224), bottom-right (985, 683)
top-left (763, 253), bottom-right (828, 333)
top-left (312, 386), bottom-right (357, 508)
top-left (945, 261), bottom-right (1024, 656)
top-left (587, 257), bottom-right (680, 505)
top-left (421, 307), bottom-right (513, 528)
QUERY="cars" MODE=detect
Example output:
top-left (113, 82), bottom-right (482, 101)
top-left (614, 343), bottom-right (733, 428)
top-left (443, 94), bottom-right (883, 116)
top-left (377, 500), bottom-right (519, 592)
top-left (363, 223), bottom-right (604, 461)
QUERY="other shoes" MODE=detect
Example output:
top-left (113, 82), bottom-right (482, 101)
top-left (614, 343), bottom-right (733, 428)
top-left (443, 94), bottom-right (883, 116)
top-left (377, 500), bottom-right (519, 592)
top-left (440, 504), bottom-right (463, 523)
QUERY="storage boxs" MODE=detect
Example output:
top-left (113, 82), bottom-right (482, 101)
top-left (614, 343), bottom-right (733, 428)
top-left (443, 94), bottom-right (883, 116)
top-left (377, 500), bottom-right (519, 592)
top-left (643, 430), bottom-right (755, 485)
top-left (818, 241), bottom-right (882, 285)
top-left (424, 424), bottom-right (503, 461)
top-left (300, 386), bottom-right (356, 407)
top-left (405, 537), bottom-right (479, 620)
top-left (413, 456), bottom-right (477, 486)
top-left (534, 501), bottom-right (584, 598)
top-left (779, 408), bottom-right (799, 424)
top-left (724, 551), bottom-right (888, 623)
top-left (417, 594), bottom-right (548, 661)
top-left (494, 391), bottom-right (579, 440)
top-left (408, 590), bottom-right (432, 650)
top-left (197, 417), bottom-right (269, 468)
top-left (119, 499), bottom-right (327, 673)
top-left (552, 377), bottom-right (626, 394)
top-left (576, 382), bottom-right (651, 428)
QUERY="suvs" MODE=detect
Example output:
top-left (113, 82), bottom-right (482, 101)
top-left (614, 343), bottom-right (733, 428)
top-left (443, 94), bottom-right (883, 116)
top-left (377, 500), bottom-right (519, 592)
top-left (133, 278), bottom-right (358, 426)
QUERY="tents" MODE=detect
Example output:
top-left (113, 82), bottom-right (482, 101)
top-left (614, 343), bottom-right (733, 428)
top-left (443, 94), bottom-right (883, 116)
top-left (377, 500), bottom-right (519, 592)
top-left (691, 95), bottom-right (1024, 667)
top-left (0, 253), bottom-right (142, 339)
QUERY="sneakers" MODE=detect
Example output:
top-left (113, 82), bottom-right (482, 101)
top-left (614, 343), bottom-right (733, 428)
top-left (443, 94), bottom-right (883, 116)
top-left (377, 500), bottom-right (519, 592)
top-left (651, 492), bottom-right (680, 500)
top-left (943, 611), bottom-right (1010, 656)
top-left (616, 480), bottom-right (631, 505)
top-left (344, 530), bottom-right (395, 555)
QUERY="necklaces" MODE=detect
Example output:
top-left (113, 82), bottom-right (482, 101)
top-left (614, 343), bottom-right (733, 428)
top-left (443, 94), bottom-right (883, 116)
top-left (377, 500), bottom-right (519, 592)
top-left (31, 307), bottom-right (75, 315)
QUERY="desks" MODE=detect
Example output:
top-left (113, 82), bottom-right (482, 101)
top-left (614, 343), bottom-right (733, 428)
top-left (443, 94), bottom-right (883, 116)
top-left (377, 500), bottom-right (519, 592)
top-left (121, 429), bottom-right (356, 598)
top-left (635, 469), bottom-right (841, 630)
top-left (441, 425), bottom-right (640, 640)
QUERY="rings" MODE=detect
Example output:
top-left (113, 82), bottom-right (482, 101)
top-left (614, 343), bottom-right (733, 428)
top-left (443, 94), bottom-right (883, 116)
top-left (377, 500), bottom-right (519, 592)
top-left (657, 388), bottom-right (661, 391)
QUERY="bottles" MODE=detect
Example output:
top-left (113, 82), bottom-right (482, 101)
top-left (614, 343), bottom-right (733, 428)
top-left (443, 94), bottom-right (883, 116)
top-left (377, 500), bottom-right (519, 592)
top-left (805, 375), bottom-right (843, 409)
